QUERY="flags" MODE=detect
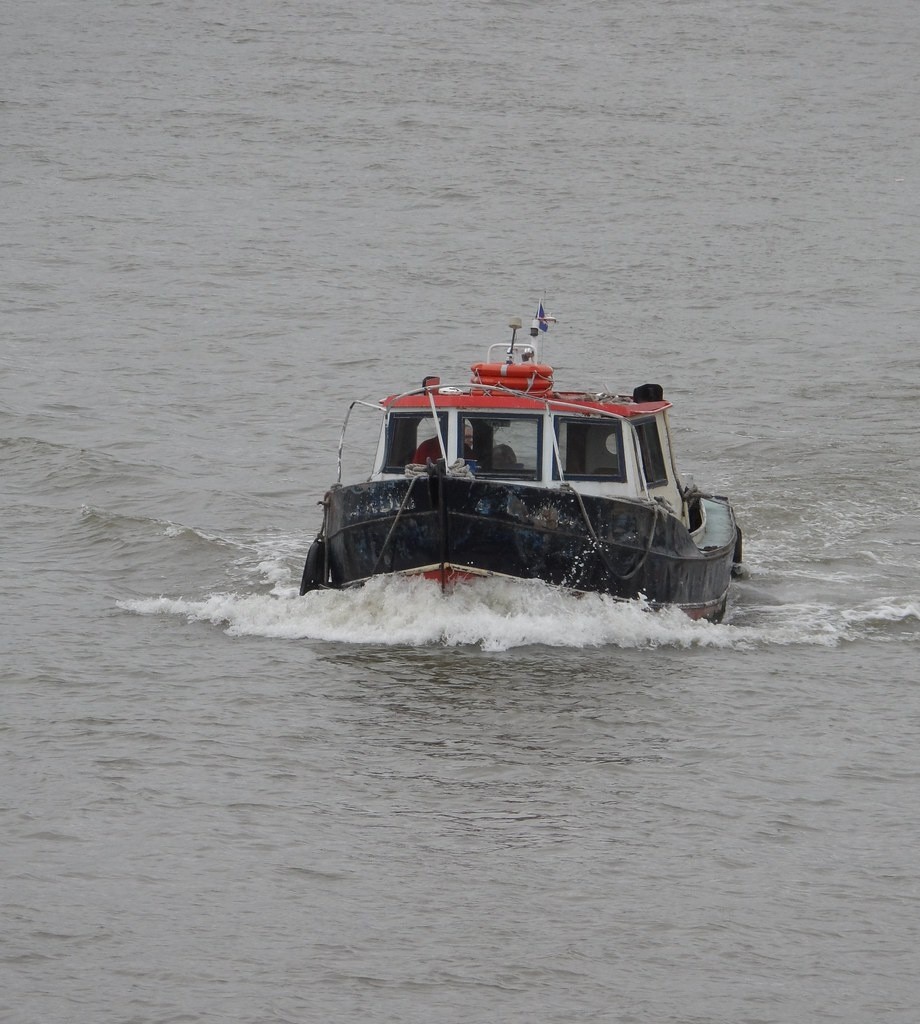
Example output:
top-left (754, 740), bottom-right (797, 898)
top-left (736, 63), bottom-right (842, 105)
top-left (535, 302), bottom-right (549, 333)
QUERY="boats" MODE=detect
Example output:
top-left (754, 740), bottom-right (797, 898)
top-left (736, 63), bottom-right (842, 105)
top-left (300, 291), bottom-right (748, 627)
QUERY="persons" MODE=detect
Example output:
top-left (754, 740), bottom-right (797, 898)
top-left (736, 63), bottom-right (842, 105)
top-left (465, 423), bottom-right (474, 460)
top-left (413, 416), bottom-right (473, 468)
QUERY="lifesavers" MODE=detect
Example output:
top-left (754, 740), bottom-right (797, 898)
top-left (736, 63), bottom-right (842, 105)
top-left (470, 362), bottom-right (553, 400)
